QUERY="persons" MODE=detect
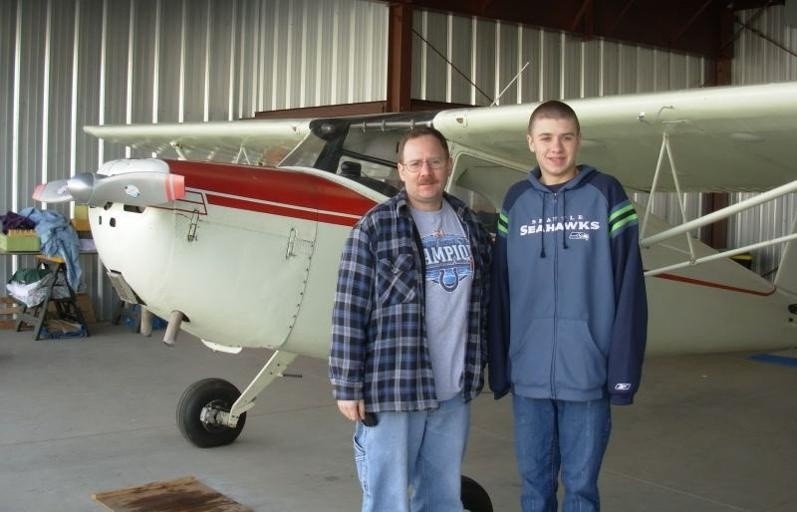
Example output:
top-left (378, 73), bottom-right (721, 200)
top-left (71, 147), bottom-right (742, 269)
top-left (329, 126), bottom-right (496, 512)
top-left (488, 99), bottom-right (648, 512)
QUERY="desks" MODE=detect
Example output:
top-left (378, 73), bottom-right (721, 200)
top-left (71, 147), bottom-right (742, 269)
top-left (0, 248), bottom-right (127, 335)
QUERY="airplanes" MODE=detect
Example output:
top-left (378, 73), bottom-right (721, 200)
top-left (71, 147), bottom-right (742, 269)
top-left (33, 78), bottom-right (797, 448)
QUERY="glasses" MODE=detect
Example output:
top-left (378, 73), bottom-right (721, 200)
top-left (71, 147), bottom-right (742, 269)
top-left (401, 157), bottom-right (448, 173)
